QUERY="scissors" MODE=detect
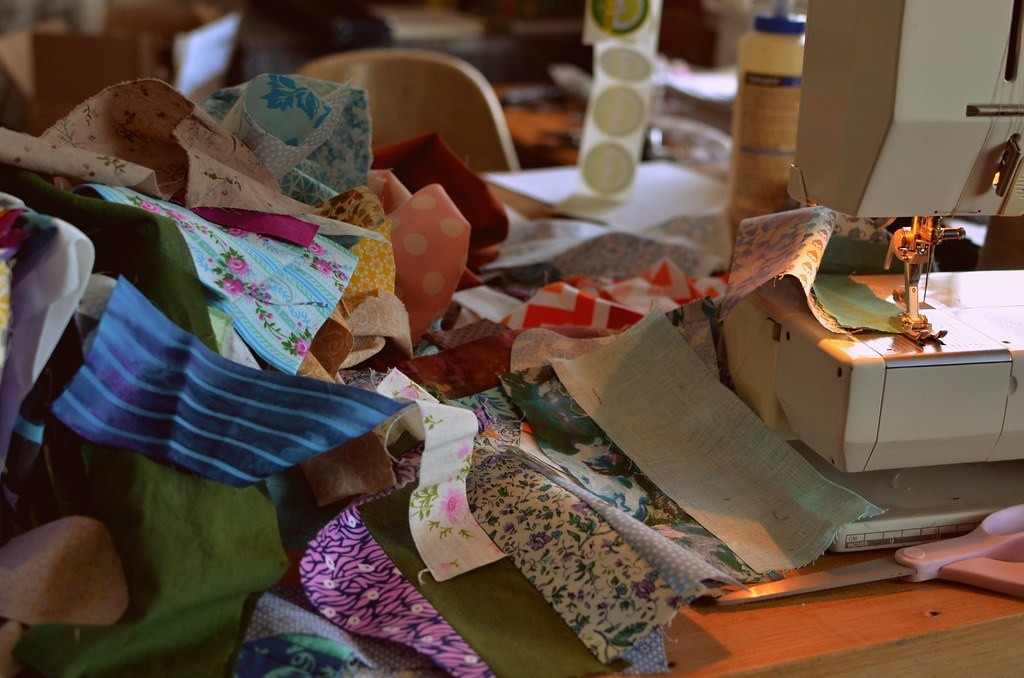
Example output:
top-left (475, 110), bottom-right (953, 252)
top-left (718, 502), bottom-right (1024, 605)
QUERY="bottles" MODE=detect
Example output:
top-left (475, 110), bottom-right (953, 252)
top-left (732, 0), bottom-right (806, 199)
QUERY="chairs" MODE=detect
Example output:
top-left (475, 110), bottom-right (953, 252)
top-left (288, 49), bottom-right (515, 172)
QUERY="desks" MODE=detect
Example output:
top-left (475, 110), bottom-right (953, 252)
top-left (89, 159), bottom-right (1024, 678)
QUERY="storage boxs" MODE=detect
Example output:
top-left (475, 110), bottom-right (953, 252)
top-left (0, 16), bottom-right (166, 134)
